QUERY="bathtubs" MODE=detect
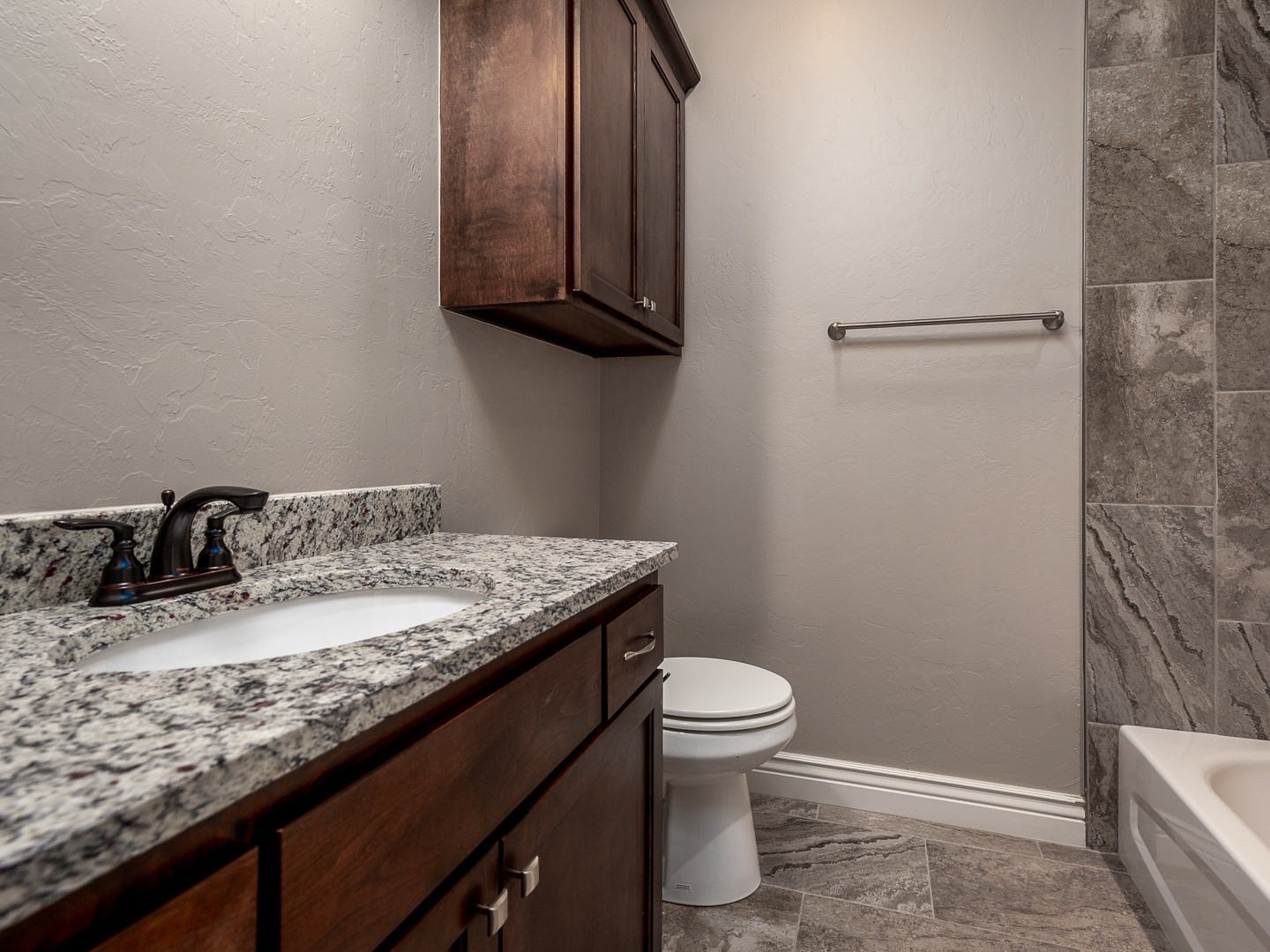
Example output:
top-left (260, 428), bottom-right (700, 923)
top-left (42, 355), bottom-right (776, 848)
top-left (1117, 724), bottom-right (1269, 952)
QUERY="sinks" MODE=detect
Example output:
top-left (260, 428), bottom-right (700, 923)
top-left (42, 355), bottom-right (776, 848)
top-left (67, 588), bottom-right (492, 673)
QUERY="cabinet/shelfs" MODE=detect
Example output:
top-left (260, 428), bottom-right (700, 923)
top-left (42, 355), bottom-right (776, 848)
top-left (0, 567), bottom-right (665, 952)
top-left (440, 0), bottom-right (700, 360)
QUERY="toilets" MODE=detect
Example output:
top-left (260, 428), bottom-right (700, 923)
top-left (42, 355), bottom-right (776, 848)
top-left (664, 657), bottom-right (797, 907)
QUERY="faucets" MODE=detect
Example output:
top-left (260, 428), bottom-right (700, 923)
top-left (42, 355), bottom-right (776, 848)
top-left (52, 484), bottom-right (270, 609)
